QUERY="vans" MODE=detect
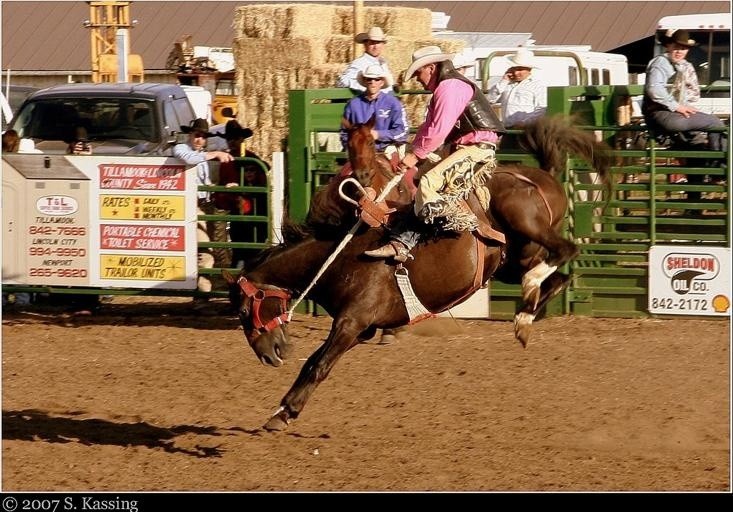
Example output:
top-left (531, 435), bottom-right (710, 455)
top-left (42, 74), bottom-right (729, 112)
top-left (451, 50), bottom-right (629, 122)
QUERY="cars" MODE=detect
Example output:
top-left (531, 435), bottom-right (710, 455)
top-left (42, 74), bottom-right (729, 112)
top-left (631, 96), bottom-right (647, 134)
top-left (1, 86), bottom-right (39, 140)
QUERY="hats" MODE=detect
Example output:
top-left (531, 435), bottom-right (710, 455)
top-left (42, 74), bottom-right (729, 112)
top-left (403, 46), bottom-right (455, 82)
top-left (504, 49), bottom-right (543, 71)
top-left (217, 120), bottom-right (253, 139)
top-left (357, 65), bottom-right (391, 89)
top-left (179, 118), bottom-right (217, 138)
top-left (353, 26), bottom-right (389, 44)
top-left (657, 29), bottom-right (697, 47)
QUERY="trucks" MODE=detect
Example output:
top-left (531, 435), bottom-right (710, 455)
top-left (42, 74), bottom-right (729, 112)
top-left (654, 13), bottom-right (729, 131)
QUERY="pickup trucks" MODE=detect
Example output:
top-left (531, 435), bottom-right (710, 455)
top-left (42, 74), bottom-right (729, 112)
top-left (5, 83), bottom-right (234, 268)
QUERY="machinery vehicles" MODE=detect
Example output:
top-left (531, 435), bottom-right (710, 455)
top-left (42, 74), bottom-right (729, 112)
top-left (83, 2), bottom-right (237, 128)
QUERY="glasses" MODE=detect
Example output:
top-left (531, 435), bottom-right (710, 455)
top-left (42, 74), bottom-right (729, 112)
top-left (365, 78), bottom-right (381, 82)
top-left (226, 136), bottom-right (243, 141)
top-left (194, 133), bottom-right (208, 140)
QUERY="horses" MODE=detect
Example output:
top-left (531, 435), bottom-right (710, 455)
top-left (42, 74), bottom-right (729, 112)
top-left (220, 109), bottom-right (614, 431)
top-left (598, 118), bottom-right (644, 217)
top-left (305, 111), bottom-right (447, 345)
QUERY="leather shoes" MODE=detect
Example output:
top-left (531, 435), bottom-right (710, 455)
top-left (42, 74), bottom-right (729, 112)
top-left (363, 242), bottom-right (408, 262)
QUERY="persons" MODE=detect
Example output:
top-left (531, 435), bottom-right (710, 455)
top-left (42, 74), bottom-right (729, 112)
top-left (1, 129), bottom-right (26, 309)
top-left (338, 64), bottom-right (409, 163)
top-left (484, 49), bottom-right (544, 130)
top-left (59, 126), bottom-right (103, 308)
top-left (170, 118), bottom-right (236, 316)
top-left (214, 120), bottom-right (272, 309)
top-left (639, 26), bottom-right (729, 217)
top-left (336, 24), bottom-right (395, 94)
top-left (362, 44), bottom-right (504, 263)
